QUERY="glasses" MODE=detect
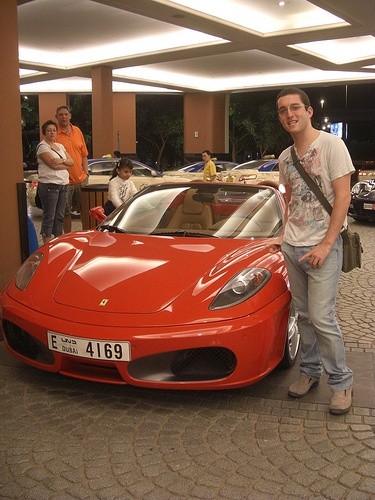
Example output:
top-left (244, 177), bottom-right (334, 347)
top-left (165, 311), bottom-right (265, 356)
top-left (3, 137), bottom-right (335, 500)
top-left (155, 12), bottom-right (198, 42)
top-left (277, 105), bottom-right (308, 116)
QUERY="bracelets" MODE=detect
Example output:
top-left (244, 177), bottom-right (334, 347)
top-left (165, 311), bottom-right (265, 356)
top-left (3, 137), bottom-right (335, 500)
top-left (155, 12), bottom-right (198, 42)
top-left (86, 174), bottom-right (89, 177)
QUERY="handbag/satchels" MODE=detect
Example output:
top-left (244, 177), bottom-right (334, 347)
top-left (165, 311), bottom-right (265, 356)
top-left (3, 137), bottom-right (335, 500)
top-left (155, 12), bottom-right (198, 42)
top-left (340, 227), bottom-right (363, 273)
top-left (35, 189), bottom-right (42, 209)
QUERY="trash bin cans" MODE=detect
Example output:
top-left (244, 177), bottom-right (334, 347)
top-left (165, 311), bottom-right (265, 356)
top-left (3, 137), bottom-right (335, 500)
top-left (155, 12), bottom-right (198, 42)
top-left (81, 184), bottom-right (109, 231)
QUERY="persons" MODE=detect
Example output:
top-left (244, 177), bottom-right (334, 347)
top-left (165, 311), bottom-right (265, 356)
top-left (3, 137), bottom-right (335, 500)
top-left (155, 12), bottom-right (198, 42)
top-left (202, 150), bottom-right (216, 182)
top-left (53, 106), bottom-right (88, 234)
top-left (278, 88), bottom-right (356, 415)
top-left (37, 120), bottom-right (74, 244)
top-left (105, 150), bottom-right (138, 216)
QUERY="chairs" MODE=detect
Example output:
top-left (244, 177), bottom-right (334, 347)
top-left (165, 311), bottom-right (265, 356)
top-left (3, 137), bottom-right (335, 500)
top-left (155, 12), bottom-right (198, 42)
top-left (246, 194), bottom-right (278, 232)
top-left (166, 189), bottom-right (213, 230)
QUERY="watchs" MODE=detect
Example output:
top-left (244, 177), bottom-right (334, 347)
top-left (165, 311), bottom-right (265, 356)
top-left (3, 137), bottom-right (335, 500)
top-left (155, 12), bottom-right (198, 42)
top-left (62, 159), bottom-right (66, 164)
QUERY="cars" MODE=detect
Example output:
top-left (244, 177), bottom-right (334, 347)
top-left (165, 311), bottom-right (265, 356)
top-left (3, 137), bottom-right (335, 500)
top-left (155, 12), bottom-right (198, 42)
top-left (0, 179), bottom-right (301, 390)
top-left (163, 158), bottom-right (239, 179)
top-left (230, 154), bottom-right (280, 182)
top-left (351, 178), bottom-right (374, 223)
top-left (28, 154), bottom-right (168, 219)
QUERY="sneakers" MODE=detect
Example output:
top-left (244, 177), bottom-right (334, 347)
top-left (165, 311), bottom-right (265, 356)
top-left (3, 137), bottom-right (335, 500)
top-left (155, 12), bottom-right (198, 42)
top-left (288, 375), bottom-right (319, 398)
top-left (329, 387), bottom-right (353, 414)
